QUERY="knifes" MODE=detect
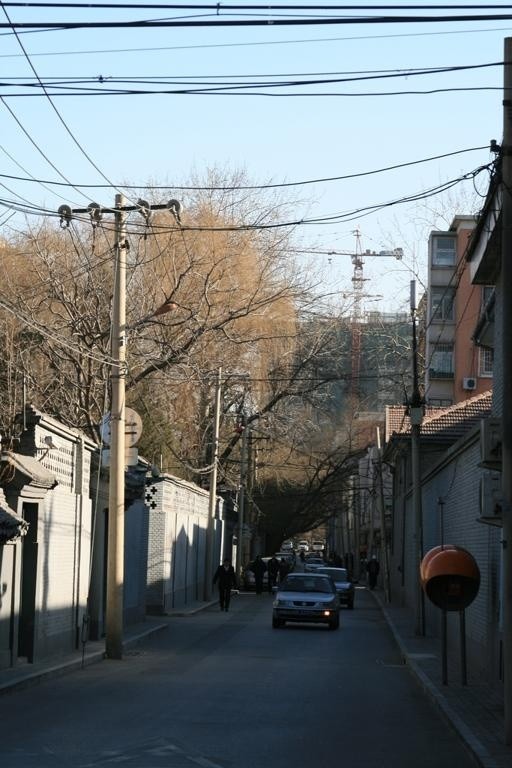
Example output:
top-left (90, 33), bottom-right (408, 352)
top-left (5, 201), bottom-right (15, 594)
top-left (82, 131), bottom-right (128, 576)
top-left (461, 377), bottom-right (477, 391)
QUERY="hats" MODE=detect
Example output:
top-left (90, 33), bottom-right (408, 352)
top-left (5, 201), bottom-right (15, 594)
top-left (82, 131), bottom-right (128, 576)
top-left (303, 558), bottom-right (328, 573)
top-left (313, 568), bottom-right (359, 609)
top-left (271, 572), bottom-right (341, 629)
top-left (244, 549), bottom-right (295, 590)
top-left (282, 541), bottom-right (293, 551)
top-left (312, 541), bottom-right (325, 552)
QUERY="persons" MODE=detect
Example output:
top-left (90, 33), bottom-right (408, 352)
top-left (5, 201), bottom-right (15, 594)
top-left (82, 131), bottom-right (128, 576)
top-left (366, 554), bottom-right (380, 591)
top-left (300, 551), bottom-right (305, 560)
top-left (280, 557), bottom-right (288, 582)
top-left (267, 552), bottom-right (279, 595)
top-left (213, 558), bottom-right (238, 611)
top-left (250, 555), bottom-right (266, 601)
